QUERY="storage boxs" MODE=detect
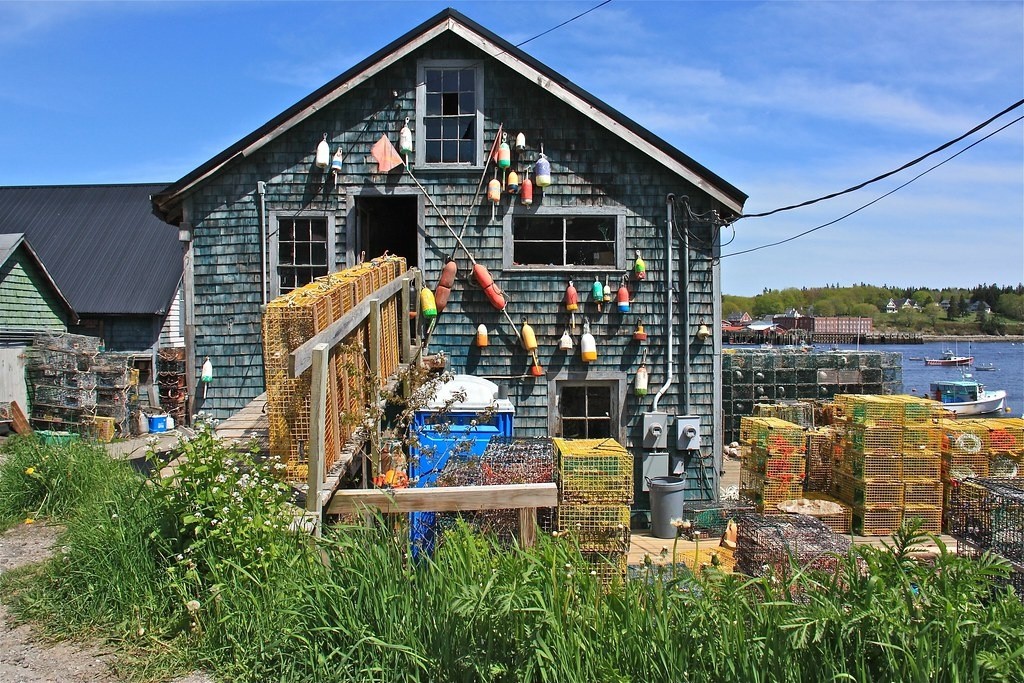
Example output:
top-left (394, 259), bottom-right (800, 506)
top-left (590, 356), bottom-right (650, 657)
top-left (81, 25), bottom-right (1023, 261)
top-left (30, 333), bottom-right (189, 449)
top-left (409, 347), bottom-right (1024, 609)
top-left (259, 254), bottom-right (407, 484)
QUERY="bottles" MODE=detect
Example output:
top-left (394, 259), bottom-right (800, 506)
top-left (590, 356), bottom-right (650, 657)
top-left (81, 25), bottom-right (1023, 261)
top-left (581, 331), bottom-right (597, 362)
top-left (522, 322), bottom-right (538, 350)
top-left (566, 281), bottom-right (578, 310)
top-left (634, 366), bottom-right (648, 395)
top-left (592, 279), bottom-right (603, 303)
top-left (635, 256), bottom-right (646, 280)
top-left (421, 287), bottom-right (437, 319)
top-left (476, 324), bottom-right (488, 347)
top-left (201, 357), bottom-right (212, 383)
top-left (603, 284), bottom-right (611, 301)
top-left (399, 125), bottom-right (413, 153)
top-left (559, 331), bottom-right (573, 349)
top-left (331, 152), bottom-right (342, 171)
top-left (617, 284), bottom-right (630, 313)
top-left (487, 132), bottom-right (552, 204)
top-left (316, 139), bottom-right (330, 168)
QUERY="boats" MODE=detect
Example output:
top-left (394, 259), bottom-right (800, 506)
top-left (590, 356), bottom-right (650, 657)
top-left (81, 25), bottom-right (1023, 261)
top-left (929, 339), bottom-right (1007, 417)
top-left (758, 332), bottom-right (838, 354)
top-left (974, 362), bottom-right (997, 372)
top-left (924, 338), bottom-right (973, 366)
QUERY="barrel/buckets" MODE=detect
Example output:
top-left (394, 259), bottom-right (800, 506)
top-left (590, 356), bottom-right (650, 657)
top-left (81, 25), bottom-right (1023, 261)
top-left (645, 472), bottom-right (687, 539)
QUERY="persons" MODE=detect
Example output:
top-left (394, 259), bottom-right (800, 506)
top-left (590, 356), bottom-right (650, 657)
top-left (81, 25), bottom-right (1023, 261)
top-left (935, 387), bottom-right (941, 401)
